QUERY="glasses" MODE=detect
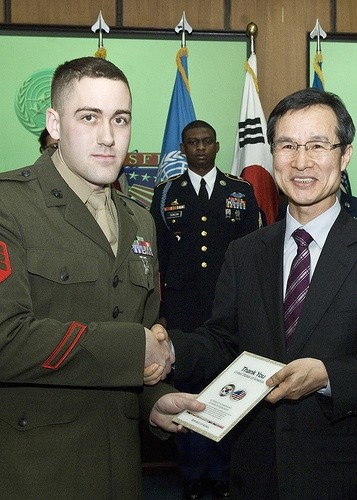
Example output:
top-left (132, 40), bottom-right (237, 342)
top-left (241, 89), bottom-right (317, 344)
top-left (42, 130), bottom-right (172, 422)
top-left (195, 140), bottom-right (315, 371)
top-left (43, 143), bottom-right (58, 149)
top-left (271, 139), bottom-right (342, 157)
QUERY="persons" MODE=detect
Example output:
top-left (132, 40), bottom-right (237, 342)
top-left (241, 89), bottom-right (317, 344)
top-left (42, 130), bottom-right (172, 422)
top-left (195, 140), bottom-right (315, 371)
top-left (150, 120), bottom-right (260, 332)
top-left (39, 127), bottom-right (59, 154)
top-left (0, 57), bottom-right (206, 500)
top-left (143, 88), bottom-right (357, 500)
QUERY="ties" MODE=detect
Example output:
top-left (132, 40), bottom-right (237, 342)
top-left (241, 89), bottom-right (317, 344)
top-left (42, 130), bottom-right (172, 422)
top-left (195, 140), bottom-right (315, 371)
top-left (283, 228), bottom-right (314, 357)
top-left (198, 178), bottom-right (209, 210)
top-left (88, 192), bottom-right (119, 259)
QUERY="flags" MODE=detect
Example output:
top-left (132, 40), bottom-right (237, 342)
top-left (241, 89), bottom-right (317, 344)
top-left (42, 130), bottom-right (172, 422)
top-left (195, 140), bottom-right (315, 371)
top-left (312, 55), bottom-right (351, 195)
top-left (155, 48), bottom-right (196, 188)
top-left (230, 55), bottom-right (283, 228)
